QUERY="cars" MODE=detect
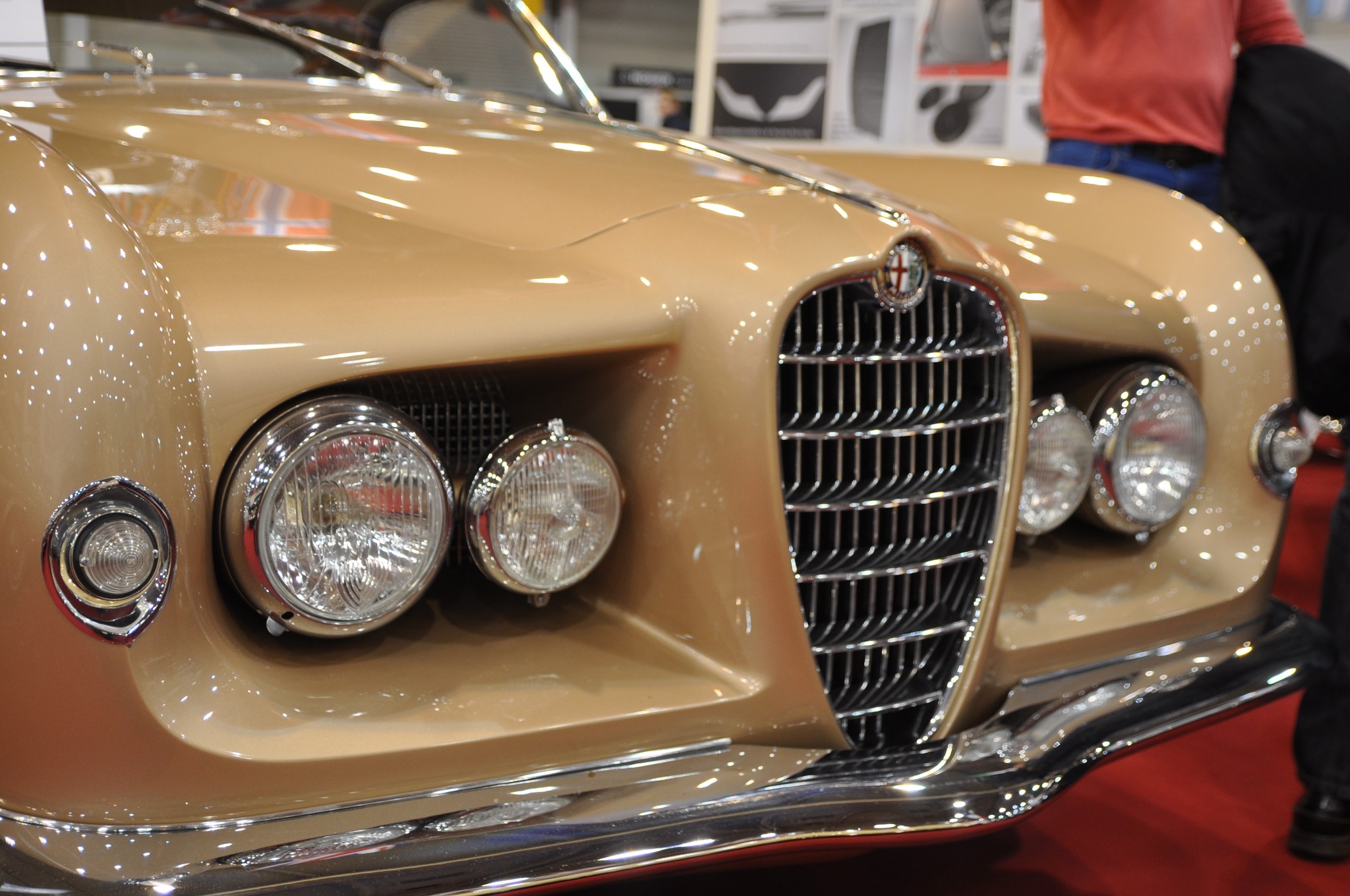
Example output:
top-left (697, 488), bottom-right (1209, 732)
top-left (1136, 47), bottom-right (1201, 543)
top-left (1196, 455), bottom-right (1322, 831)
top-left (0, 0), bottom-right (1337, 896)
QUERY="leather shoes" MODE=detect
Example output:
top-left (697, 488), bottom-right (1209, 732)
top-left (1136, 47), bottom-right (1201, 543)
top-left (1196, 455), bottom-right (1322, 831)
top-left (1287, 791), bottom-right (1350, 865)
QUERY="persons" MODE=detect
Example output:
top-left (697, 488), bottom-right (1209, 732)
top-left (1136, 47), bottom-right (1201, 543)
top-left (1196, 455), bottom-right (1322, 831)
top-left (1039, 0), bottom-right (1308, 204)
top-left (1285, 428), bottom-right (1349, 862)
